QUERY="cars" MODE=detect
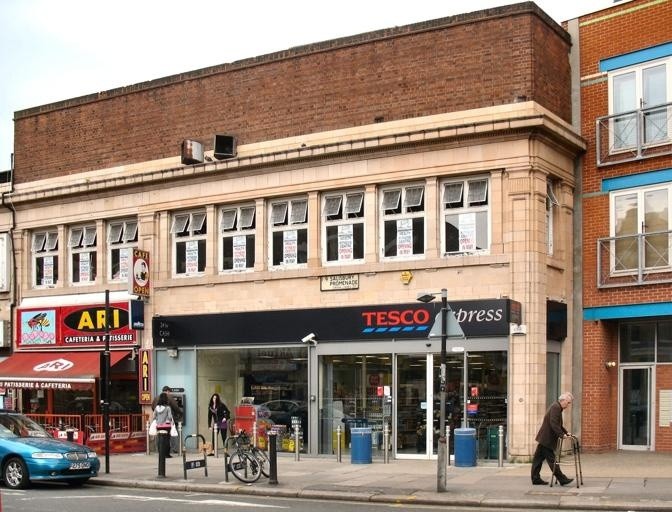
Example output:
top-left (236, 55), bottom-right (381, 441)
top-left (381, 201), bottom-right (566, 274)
top-left (260, 400), bottom-right (363, 445)
top-left (0, 412), bottom-right (100, 490)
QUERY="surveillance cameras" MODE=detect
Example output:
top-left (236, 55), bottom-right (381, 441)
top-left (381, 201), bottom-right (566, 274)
top-left (301, 333), bottom-right (315, 343)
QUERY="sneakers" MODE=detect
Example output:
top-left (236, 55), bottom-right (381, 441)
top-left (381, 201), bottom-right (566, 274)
top-left (534, 482), bottom-right (548, 485)
top-left (561, 479), bottom-right (573, 486)
top-left (207, 453), bottom-right (214, 456)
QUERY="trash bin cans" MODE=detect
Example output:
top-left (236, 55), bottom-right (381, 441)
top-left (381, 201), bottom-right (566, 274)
top-left (453, 427), bottom-right (477, 467)
top-left (350, 427), bottom-right (372, 464)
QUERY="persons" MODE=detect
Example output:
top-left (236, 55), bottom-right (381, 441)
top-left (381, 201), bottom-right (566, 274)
top-left (140, 261), bottom-right (149, 281)
top-left (152, 393), bottom-right (173, 459)
top-left (207, 393), bottom-right (231, 457)
top-left (151, 386), bottom-right (183, 453)
top-left (529, 390), bottom-right (575, 487)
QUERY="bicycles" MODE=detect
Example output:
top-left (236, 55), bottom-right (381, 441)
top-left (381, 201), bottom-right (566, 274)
top-left (228, 429), bottom-right (270, 482)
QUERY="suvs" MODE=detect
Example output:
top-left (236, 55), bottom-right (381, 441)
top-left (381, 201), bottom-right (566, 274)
top-left (67, 397), bottom-right (126, 415)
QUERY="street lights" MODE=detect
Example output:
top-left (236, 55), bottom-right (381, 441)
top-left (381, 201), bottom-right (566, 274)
top-left (417, 288), bottom-right (447, 491)
